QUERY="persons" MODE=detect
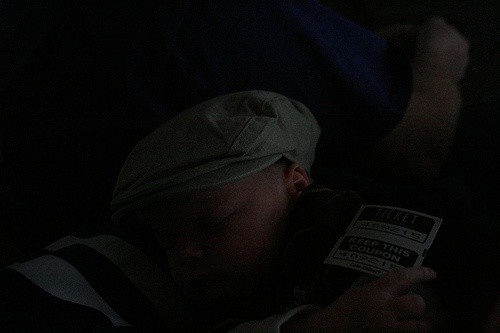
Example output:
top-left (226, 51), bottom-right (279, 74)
top-left (103, 98), bottom-right (496, 332)
top-left (150, 1), bottom-right (472, 155)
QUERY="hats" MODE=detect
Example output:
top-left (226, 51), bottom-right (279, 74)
top-left (107, 89), bottom-right (322, 221)
